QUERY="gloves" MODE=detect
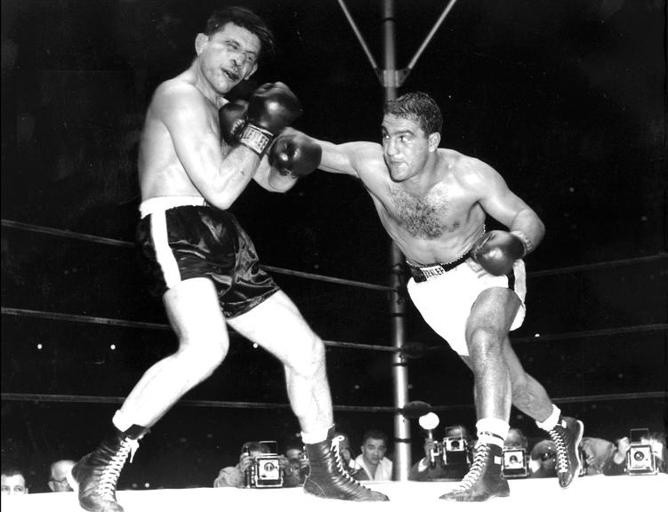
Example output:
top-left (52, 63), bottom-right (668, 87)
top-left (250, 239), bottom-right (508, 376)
top-left (236, 81), bottom-right (304, 160)
top-left (267, 136), bottom-right (322, 180)
top-left (220, 97), bottom-right (249, 148)
top-left (469, 228), bottom-right (533, 276)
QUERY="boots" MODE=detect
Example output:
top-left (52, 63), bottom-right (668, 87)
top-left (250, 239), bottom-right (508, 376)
top-left (301, 424), bottom-right (389, 502)
top-left (545, 414), bottom-right (585, 489)
top-left (440, 443), bottom-right (510, 502)
top-left (66, 422), bottom-right (147, 512)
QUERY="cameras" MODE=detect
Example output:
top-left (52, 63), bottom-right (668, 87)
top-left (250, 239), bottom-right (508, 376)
top-left (444, 436), bottom-right (465, 461)
top-left (255, 454), bottom-right (282, 487)
top-left (502, 449), bottom-right (525, 478)
top-left (623, 445), bottom-right (654, 473)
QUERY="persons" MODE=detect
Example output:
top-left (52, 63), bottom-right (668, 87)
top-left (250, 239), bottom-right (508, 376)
top-left (213, 442), bottom-right (307, 488)
top-left (409, 424), bottom-right (476, 480)
top-left (48, 461), bottom-right (76, 492)
top-left (332, 433), bottom-right (392, 482)
top-left (1, 470), bottom-right (29, 492)
top-left (219, 90), bottom-right (583, 501)
top-left (501, 429), bottom-right (667, 477)
top-left (67, 6), bottom-right (387, 512)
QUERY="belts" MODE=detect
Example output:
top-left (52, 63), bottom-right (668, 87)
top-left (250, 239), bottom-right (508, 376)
top-left (406, 252), bottom-right (471, 283)
